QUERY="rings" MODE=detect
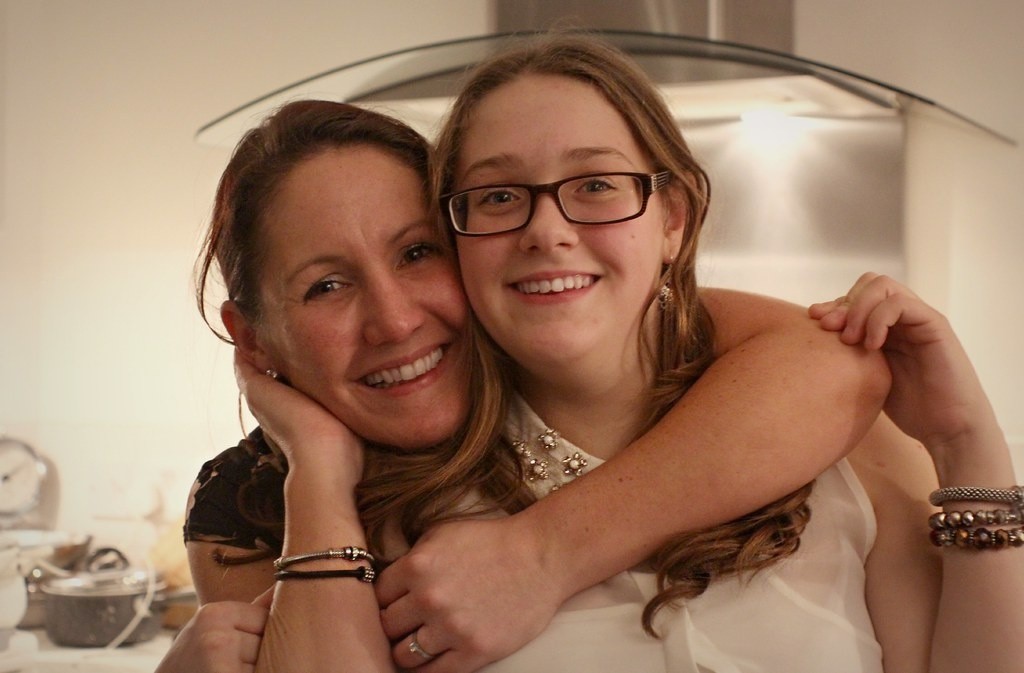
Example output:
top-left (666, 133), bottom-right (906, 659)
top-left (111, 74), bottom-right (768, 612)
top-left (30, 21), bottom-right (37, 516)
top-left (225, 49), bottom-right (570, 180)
top-left (409, 630), bottom-right (432, 659)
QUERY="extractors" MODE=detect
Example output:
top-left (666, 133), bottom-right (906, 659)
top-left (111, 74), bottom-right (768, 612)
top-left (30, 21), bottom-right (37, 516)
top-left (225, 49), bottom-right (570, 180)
top-left (194, 0), bottom-right (1020, 150)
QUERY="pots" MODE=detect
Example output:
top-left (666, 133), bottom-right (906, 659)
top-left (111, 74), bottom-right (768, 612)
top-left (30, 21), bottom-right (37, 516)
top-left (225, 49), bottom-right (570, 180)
top-left (40, 547), bottom-right (199, 648)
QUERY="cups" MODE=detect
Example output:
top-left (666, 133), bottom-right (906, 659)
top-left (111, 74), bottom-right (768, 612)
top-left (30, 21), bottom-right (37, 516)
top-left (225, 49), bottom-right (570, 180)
top-left (0, 546), bottom-right (27, 648)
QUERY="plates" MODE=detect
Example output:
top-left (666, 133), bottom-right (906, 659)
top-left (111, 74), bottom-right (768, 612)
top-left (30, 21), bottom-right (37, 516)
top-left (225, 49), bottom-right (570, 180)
top-left (0, 524), bottom-right (92, 573)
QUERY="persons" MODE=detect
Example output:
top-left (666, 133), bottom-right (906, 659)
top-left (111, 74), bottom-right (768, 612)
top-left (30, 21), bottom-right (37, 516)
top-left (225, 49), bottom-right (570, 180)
top-left (155, 101), bottom-right (893, 672)
top-left (254, 38), bottom-right (1023, 673)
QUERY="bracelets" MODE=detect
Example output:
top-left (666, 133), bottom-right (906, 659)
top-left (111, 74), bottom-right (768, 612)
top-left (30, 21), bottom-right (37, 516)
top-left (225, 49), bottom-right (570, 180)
top-left (274, 546), bottom-right (378, 568)
top-left (276, 565), bottom-right (377, 583)
top-left (928, 487), bottom-right (1024, 550)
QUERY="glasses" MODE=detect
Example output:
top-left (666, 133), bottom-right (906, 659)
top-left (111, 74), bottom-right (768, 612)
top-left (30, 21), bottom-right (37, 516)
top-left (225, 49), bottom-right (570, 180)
top-left (439, 169), bottom-right (673, 237)
